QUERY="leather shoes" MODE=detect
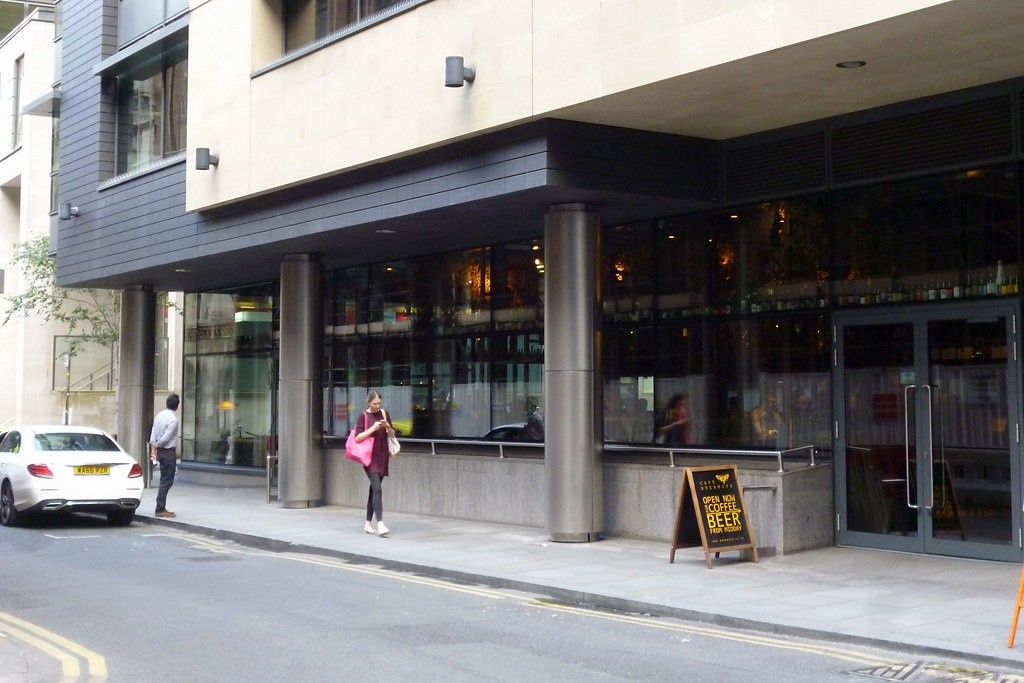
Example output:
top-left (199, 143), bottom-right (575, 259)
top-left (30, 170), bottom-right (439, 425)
top-left (155, 508), bottom-right (176, 518)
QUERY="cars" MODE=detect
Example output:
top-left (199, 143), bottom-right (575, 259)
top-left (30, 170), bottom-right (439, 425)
top-left (0, 425), bottom-right (144, 520)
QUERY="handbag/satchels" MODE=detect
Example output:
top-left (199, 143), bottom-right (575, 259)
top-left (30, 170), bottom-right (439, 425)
top-left (345, 410), bottom-right (375, 467)
top-left (647, 409), bottom-right (671, 445)
top-left (379, 408), bottom-right (401, 456)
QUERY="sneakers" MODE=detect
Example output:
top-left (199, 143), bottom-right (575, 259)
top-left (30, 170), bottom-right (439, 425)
top-left (378, 524), bottom-right (390, 537)
top-left (363, 524), bottom-right (377, 534)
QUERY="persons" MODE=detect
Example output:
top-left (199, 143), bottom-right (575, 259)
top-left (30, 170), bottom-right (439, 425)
top-left (750, 391), bottom-right (784, 445)
top-left (147, 393), bottom-right (180, 518)
top-left (353, 389), bottom-right (397, 537)
top-left (504, 384), bottom-right (649, 426)
top-left (653, 392), bottom-right (692, 449)
